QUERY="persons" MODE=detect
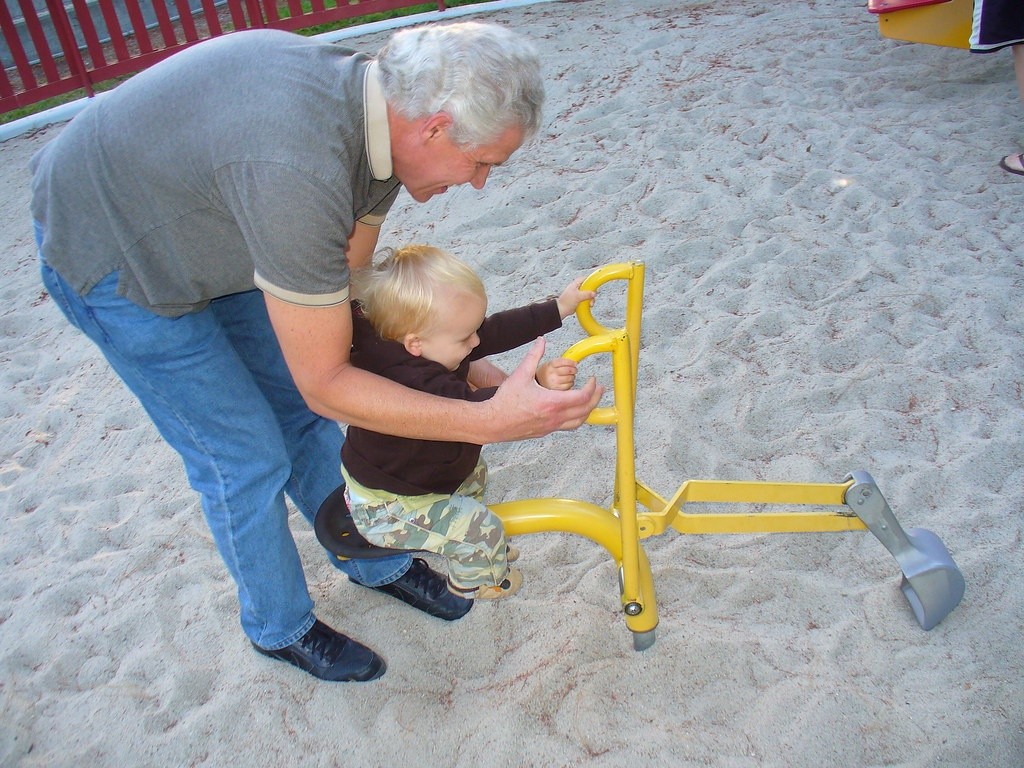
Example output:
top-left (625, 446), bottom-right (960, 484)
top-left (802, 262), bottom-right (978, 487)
top-left (21, 16), bottom-right (608, 682)
top-left (341, 238), bottom-right (601, 608)
top-left (966, 0), bottom-right (1024, 177)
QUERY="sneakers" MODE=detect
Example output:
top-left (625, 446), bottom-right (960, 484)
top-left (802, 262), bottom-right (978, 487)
top-left (253, 619), bottom-right (387, 683)
top-left (348, 554), bottom-right (475, 621)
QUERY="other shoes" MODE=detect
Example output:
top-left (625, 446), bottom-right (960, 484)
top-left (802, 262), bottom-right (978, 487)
top-left (446, 542), bottom-right (523, 598)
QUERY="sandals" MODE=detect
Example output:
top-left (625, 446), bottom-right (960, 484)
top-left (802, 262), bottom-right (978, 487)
top-left (999, 150), bottom-right (1024, 175)
